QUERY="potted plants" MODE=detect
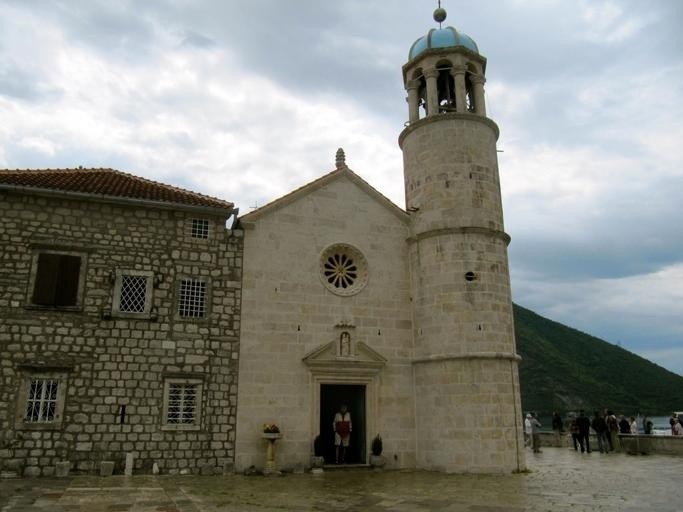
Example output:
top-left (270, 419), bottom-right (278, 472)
top-left (368, 433), bottom-right (384, 466)
top-left (311, 435), bottom-right (325, 467)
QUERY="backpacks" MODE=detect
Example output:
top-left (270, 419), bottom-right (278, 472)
top-left (615, 423), bottom-right (620, 434)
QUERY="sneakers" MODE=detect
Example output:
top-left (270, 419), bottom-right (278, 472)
top-left (525, 442), bottom-right (543, 454)
top-left (575, 448), bottom-right (617, 455)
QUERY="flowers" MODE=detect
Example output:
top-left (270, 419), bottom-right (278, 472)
top-left (262, 422), bottom-right (280, 432)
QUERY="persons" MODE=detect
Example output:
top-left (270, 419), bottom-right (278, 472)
top-left (330, 403), bottom-right (353, 464)
top-left (523, 407), bottom-right (683, 453)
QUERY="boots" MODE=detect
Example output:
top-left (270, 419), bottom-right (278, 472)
top-left (335, 445), bottom-right (339, 465)
top-left (341, 445), bottom-right (348, 464)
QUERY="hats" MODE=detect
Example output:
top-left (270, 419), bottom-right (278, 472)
top-left (526, 414), bottom-right (532, 418)
